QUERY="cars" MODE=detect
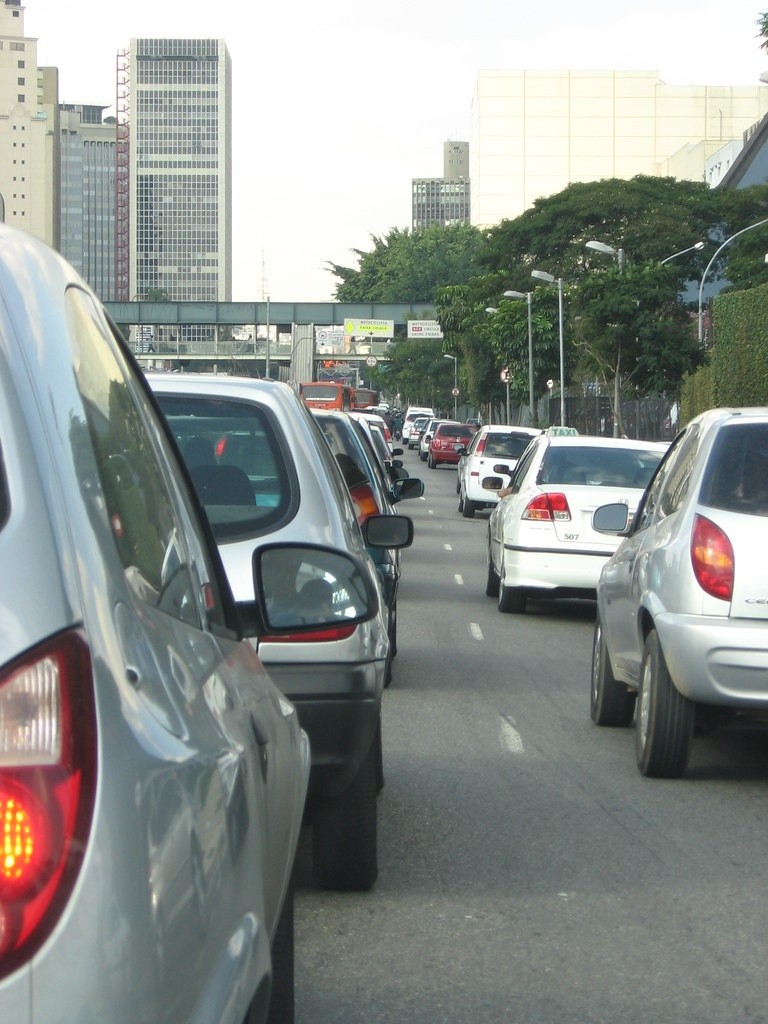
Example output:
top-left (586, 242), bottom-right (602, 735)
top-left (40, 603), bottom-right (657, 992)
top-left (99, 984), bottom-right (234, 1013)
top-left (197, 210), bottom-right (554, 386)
top-left (162, 370), bottom-right (480, 687)
top-left (454, 424), bottom-right (552, 519)
top-left (484, 434), bottom-right (672, 614)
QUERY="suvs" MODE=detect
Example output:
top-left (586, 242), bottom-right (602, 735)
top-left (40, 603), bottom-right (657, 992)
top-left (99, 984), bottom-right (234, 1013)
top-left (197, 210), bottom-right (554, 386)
top-left (140, 368), bottom-right (416, 896)
top-left (0, 218), bottom-right (387, 1024)
top-left (589, 403), bottom-right (768, 785)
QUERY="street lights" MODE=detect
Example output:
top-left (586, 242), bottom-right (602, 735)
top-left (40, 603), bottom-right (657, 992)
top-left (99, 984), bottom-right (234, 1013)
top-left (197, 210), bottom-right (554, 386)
top-left (530, 269), bottom-right (567, 429)
top-left (503, 290), bottom-right (534, 427)
top-left (443, 354), bottom-right (457, 421)
top-left (584, 239), bottom-right (625, 439)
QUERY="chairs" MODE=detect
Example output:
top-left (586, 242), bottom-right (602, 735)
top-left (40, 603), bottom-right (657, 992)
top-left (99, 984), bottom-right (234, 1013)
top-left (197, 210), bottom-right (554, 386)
top-left (633, 467), bottom-right (659, 486)
top-left (548, 464), bottom-right (586, 484)
top-left (187, 464), bottom-right (255, 506)
